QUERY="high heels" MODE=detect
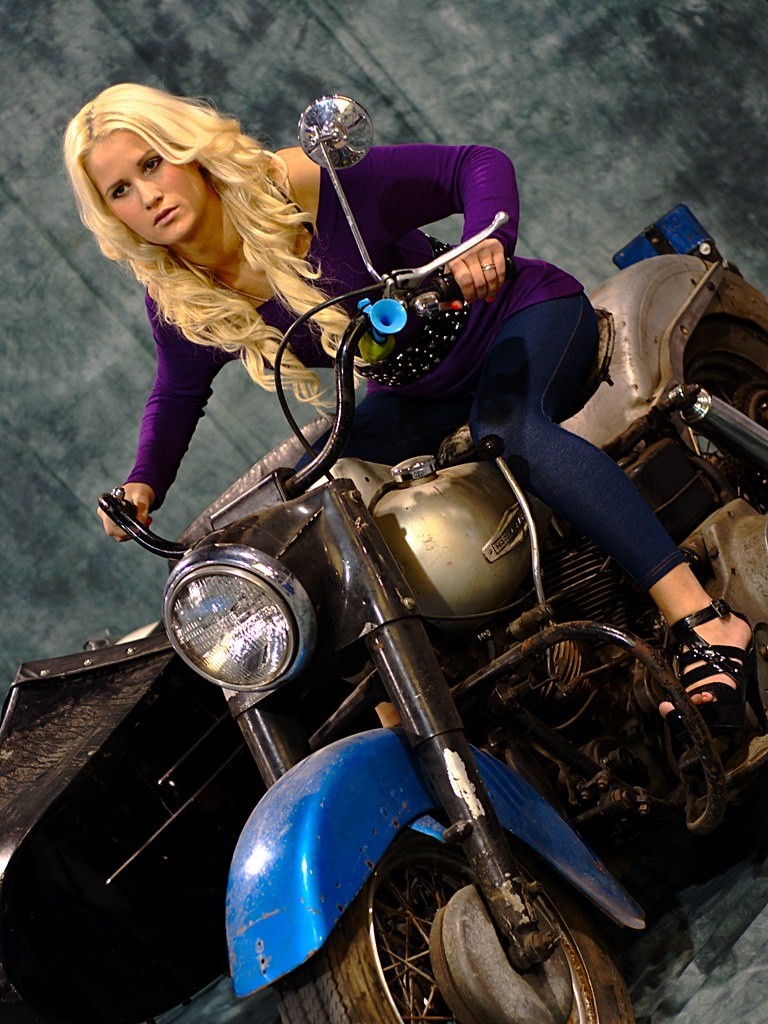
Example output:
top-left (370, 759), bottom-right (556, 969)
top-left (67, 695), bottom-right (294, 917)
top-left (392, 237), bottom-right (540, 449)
top-left (665, 598), bottom-right (767, 741)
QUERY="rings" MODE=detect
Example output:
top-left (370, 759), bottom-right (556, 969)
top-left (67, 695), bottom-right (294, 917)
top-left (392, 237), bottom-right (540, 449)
top-left (481, 263), bottom-right (495, 271)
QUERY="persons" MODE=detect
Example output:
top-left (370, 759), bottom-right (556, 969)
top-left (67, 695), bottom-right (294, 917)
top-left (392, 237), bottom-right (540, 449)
top-left (61, 80), bottom-right (766, 729)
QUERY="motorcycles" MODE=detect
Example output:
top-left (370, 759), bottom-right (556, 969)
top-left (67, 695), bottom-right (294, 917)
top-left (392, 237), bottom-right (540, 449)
top-left (97, 94), bottom-right (768, 1024)
